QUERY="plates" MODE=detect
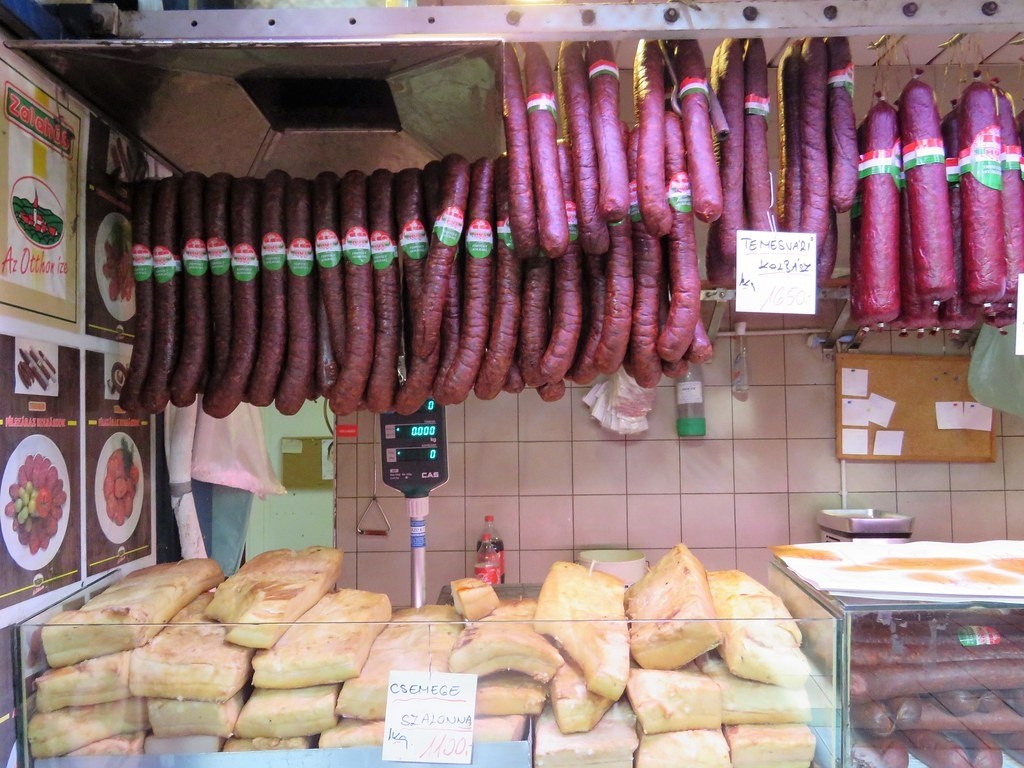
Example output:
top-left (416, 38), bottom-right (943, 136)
top-left (0, 434), bottom-right (71, 571)
top-left (96, 212), bottom-right (136, 322)
top-left (94, 431), bottom-right (144, 545)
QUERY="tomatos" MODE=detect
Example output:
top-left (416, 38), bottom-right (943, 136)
top-left (36, 488), bottom-right (51, 519)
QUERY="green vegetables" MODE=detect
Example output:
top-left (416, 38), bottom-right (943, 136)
top-left (120, 437), bottom-right (136, 475)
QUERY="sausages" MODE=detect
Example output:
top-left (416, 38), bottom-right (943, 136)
top-left (104, 449), bottom-right (139, 524)
top-left (18, 348), bottom-right (56, 391)
top-left (844, 609), bottom-right (1024, 768)
top-left (4, 453), bottom-right (67, 554)
top-left (116, 36), bottom-right (1023, 410)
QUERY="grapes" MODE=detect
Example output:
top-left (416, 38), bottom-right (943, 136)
top-left (13, 481), bottom-right (39, 533)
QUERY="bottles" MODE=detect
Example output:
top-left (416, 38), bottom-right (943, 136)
top-left (474, 534), bottom-right (499, 584)
top-left (675, 363), bottom-right (706, 437)
top-left (477, 515), bottom-right (505, 584)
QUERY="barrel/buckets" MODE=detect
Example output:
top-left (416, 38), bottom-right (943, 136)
top-left (573, 550), bottom-right (651, 591)
